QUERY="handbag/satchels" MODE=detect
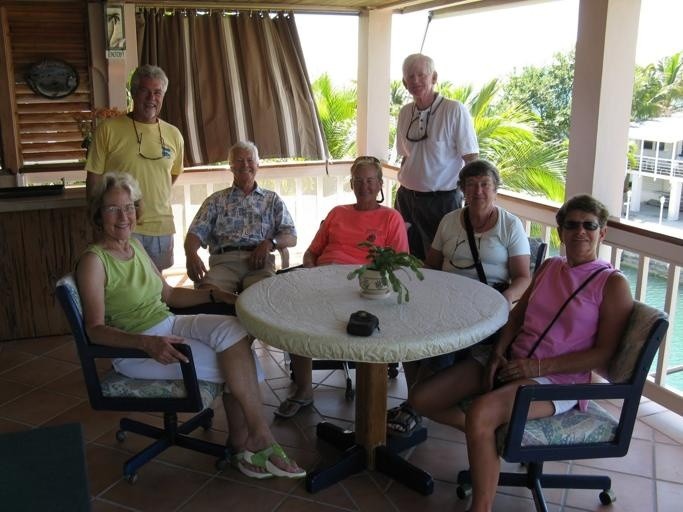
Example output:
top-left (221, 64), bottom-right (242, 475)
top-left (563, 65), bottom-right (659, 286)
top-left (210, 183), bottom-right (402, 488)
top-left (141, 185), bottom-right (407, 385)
top-left (482, 282), bottom-right (510, 346)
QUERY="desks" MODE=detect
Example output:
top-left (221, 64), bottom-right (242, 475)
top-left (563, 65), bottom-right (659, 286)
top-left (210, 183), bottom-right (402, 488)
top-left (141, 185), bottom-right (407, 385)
top-left (234, 264), bottom-right (511, 496)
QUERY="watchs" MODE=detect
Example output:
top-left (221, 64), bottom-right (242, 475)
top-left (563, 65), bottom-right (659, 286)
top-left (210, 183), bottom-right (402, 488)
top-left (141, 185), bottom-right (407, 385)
top-left (268, 236), bottom-right (278, 252)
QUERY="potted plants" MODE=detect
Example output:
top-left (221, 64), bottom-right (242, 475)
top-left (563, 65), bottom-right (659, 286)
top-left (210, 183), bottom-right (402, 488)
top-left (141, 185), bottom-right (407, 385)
top-left (345, 241), bottom-right (425, 306)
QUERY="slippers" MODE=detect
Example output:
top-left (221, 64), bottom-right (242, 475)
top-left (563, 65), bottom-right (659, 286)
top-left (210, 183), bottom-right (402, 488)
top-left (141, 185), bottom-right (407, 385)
top-left (224, 438), bottom-right (307, 479)
top-left (273, 398), bottom-right (313, 418)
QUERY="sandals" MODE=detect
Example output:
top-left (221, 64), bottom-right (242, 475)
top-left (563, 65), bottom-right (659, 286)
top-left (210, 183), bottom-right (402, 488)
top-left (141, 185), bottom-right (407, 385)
top-left (386, 401), bottom-right (423, 437)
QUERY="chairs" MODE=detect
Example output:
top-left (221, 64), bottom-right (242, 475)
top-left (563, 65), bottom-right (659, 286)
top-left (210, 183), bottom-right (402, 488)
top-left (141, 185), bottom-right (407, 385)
top-left (193, 249), bottom-right (289, 290)
top-left (55, 272), bottom-right (227, 485)
top-left (457, 301), bottom-right (669, 512)
top-left (275, 220), bottom-right (413, 403)
top-left (530, 239), bottom-right (548, 276)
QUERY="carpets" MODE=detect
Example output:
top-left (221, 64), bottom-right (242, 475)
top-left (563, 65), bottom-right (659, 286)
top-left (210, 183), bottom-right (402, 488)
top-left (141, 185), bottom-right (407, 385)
top-left (0, 422), bottom-right (91, 510)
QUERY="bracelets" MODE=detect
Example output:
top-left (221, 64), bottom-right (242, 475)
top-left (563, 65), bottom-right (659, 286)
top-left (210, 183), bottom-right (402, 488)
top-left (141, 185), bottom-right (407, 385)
top-left (208, 288), bottom-right (216, 304)
top-left (538, 359), bottom-right (541, 377)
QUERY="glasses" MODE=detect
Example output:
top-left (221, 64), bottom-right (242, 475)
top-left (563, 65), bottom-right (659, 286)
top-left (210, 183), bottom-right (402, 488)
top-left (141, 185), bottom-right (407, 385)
top-left (406, 112), bottom-right (429, 141)
top-left (100, 207), bottom-right (140, 213)
top-left (354, 156), bottom-right (381, 162)
top-left (562, 221), bottom-right (602, 230)
top-left (139, 133), bottom-right (164, 159)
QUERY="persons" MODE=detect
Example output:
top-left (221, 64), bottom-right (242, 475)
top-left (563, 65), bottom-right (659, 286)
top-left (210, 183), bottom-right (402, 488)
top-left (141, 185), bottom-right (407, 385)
top-left (386, 159), bottom-right (532, 437)
top-left (86, 63), bottom-right (185, 276)
top-left (408, 192), bottom-right (635, 512)
top-left (184, 140), bottom-right (298, 295)
top-left (394, 54), bottom-right (479, 271)
top-left (273, 158), bottom-right (410, 418)
top-left (74, 170), bottom-right (307, 480)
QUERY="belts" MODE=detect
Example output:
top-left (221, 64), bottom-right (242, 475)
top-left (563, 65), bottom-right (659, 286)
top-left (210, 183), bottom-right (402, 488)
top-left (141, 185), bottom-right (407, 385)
top-left (214, 246), bottom-right (257, 253)
